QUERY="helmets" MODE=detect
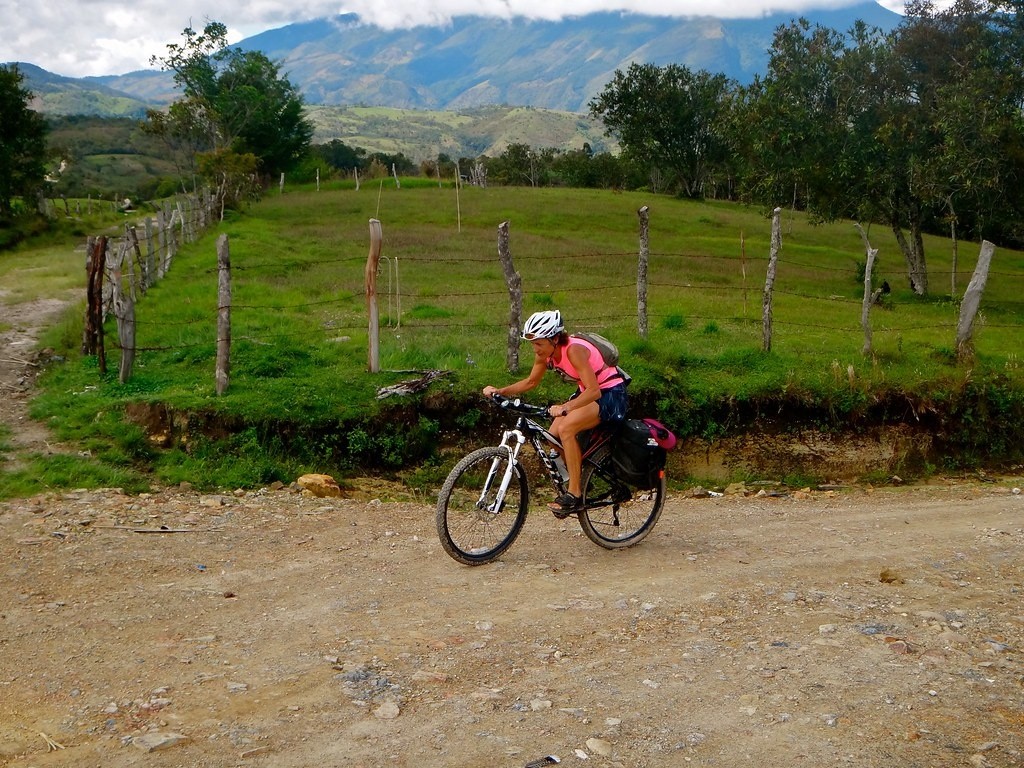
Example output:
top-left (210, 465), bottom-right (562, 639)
top-left (520, 310), bottom-right (564, 340)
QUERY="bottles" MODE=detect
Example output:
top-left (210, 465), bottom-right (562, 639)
top-left (549, 448), bottom-right (570, 483)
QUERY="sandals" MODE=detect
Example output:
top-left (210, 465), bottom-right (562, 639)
top-left (585, 482), bottom-right (595, 494)
top-left (546, 490), bottom-right (584, 514)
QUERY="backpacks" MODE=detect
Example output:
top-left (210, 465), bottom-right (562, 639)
top-left (608, 419), bottom-right (667, 488)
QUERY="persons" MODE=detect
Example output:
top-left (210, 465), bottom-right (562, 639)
top-left (481, 311), bottom-right (631, 517)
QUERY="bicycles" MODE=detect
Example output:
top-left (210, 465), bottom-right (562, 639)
top-left (435, 394), bottom-right (671, 566)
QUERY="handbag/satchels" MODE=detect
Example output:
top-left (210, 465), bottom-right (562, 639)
top-left (570, 331), bottom-right (619, 367)
